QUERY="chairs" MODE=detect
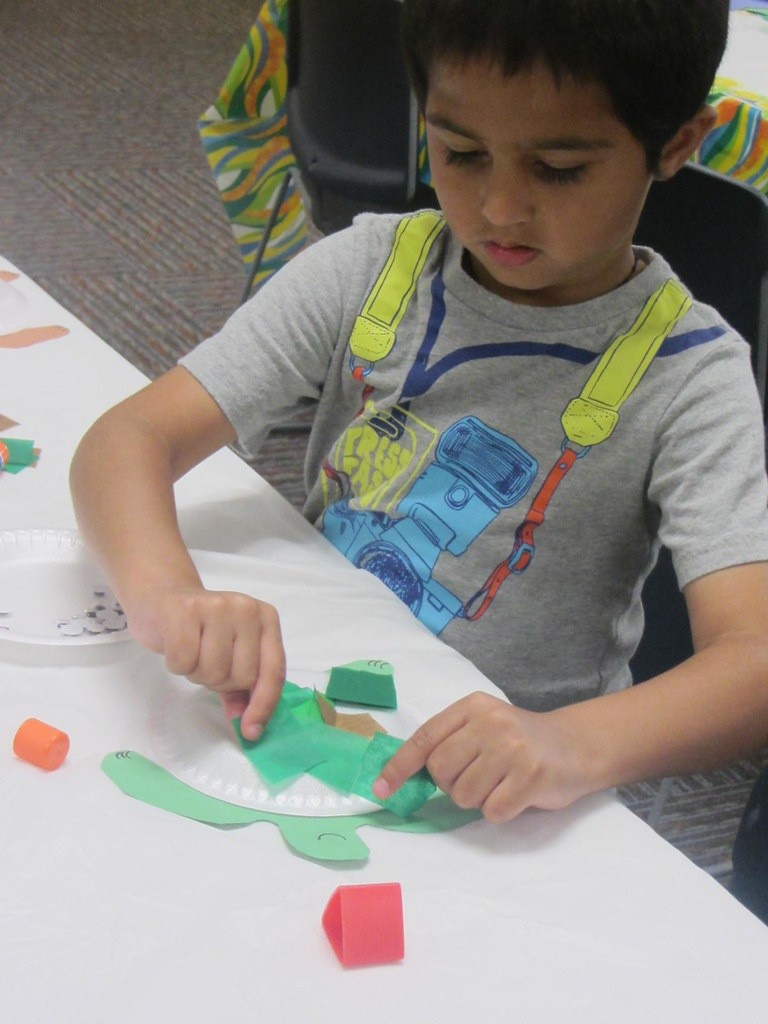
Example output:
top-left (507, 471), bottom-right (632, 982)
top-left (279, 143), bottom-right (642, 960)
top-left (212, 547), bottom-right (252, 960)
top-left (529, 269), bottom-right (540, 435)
top-left (240, 0), bottom-right (768, 439)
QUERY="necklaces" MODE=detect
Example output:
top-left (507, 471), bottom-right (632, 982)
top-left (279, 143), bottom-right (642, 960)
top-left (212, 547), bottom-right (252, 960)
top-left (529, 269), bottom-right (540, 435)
top-left (457, 248), bottom-right (639, 289)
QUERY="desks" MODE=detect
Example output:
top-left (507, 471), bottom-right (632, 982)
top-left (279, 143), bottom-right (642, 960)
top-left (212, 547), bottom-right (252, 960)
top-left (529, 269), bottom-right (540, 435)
top-left (0, 256), bottom-right (767, 1024)
top-left (685, 8), bottom-right (768, 205)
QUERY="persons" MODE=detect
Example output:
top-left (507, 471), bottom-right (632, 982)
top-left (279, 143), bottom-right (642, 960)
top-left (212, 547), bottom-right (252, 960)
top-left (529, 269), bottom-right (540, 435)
top-left (69, 0), bottom-right (768, 825)
